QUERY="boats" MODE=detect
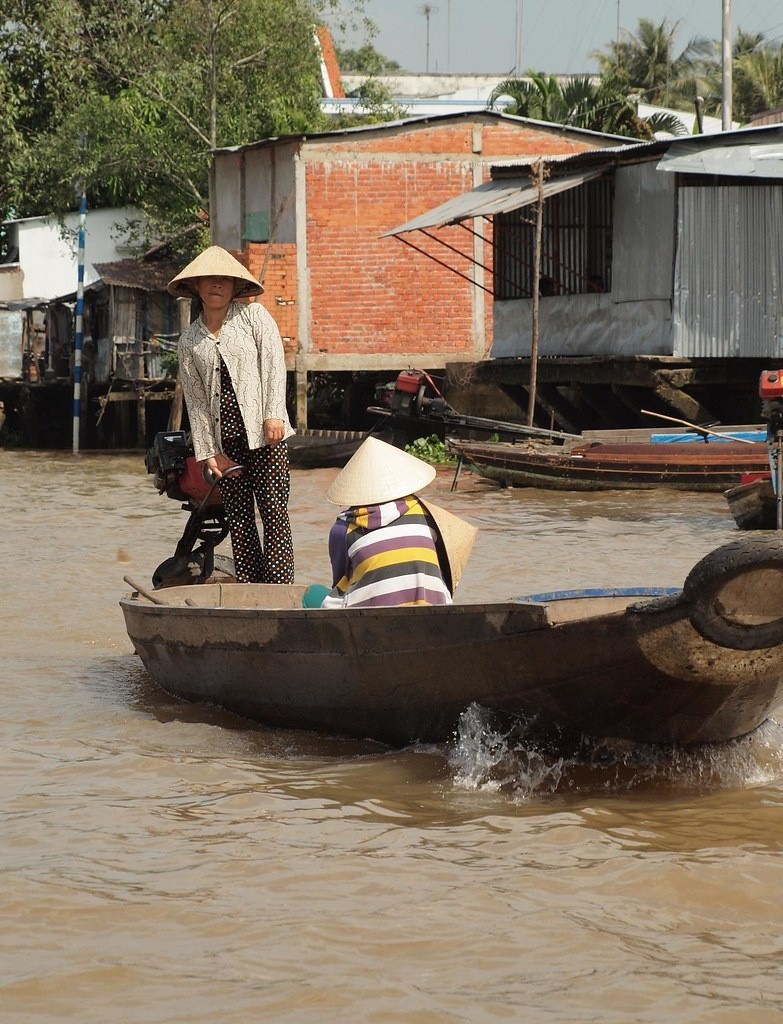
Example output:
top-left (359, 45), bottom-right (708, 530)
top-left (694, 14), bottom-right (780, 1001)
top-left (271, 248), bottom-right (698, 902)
top-left (445, 369), bottom-right (783, 493)
top-left (286, 426), bottom-right (419, 469)
top-left (367, 365), bottom-right (591, 445)
top-left (118, 432), bottom-right (783, 750)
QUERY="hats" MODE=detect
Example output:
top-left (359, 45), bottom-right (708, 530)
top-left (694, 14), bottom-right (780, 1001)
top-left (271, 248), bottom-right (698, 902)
top-left (413, 495), bottom-right (478, 601)
top-left (326, 436), bottom-right (436, 505)
top-left (168, 244), bottom-right (265, 297)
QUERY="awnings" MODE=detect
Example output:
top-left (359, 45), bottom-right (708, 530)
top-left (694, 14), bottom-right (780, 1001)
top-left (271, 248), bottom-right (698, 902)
top-left (376, 167), bottom-right (606, 299)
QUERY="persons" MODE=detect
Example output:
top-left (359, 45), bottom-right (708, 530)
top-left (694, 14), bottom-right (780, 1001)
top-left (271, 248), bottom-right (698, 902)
top-left (167, 246), bottom-right (296, 585)
top-left (302, 435), bottom-right (454, 608)
